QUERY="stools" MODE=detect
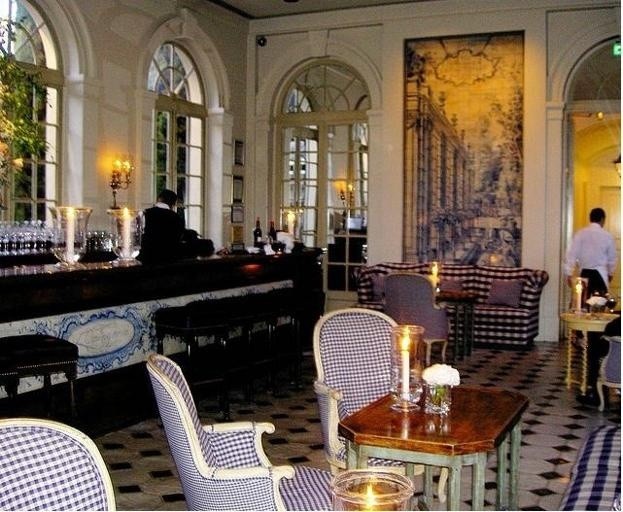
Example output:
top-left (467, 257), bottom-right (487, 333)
top-left (0, 334), bottom-right (79, 423)
top-left (152, 281), bottom-right (325, 421)
top-left (561, 424), bottom-right (620, 511)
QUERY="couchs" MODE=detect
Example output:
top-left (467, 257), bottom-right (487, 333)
top-left (349, 261), bottom-right (549, 353)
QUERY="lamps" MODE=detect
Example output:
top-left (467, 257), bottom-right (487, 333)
top-left (108, 159), bottom-right (135, 211)
top-left (334, 179), bottom-right (354, 210)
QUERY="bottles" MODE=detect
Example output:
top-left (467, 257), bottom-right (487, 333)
top-left (252, 218), bottom-right (294, 255)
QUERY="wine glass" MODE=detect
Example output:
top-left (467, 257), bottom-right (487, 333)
top-left (588, 290), bottom-right (617, 320)
top-left (0, 206), bottom-right (144, 272)
top-left (386, 324), bottom-right (422, 413)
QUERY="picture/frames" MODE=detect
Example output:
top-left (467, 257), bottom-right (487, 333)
top-left (231, 205), bottom-right (244, 223)
top-left (230, 175), bottom-right (244, 203)
top-left (231, 139), bottom-right (244, 167)
top-left (230, 225), bottom-right (244, 243)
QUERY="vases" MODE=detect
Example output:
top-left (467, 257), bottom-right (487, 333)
top-left (424, 383), bottom-right (451, 413)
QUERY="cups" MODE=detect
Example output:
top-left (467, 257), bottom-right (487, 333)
top-left (328, 469), bottom-right (414, 511)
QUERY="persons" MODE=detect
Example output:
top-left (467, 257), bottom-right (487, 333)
top-left (133, 189), bottom-right (184, 260)
top-left (566, 207), bottom-right (615, 350)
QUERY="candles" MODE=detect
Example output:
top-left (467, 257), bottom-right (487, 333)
top-left (400, 329), bottom-right (410, 392)
top-left (431, 263), bottom-right (438, 283)
top-left (64, 207), bottom-right (74, 258)
top-left (121, 207), bottom-right (130, 253)
top-left (575, 283), bottom-right (583, 308)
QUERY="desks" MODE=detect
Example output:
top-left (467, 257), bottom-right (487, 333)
top-left (560, 311), bottom-right (621, 396)
top-left (433, 289), bottom-right (488, 360)
top-left (335, 383), bottom-right (530, 511)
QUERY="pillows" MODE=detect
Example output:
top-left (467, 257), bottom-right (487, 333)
top-left (371, 273), bottom-right (384, 296)
top-left (439, 278), bottom-right (463, 289)
top-left (486, 280), bottom-right (523, 309)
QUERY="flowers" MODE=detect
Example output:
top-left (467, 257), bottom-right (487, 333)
top-left (420, 363), bottom-right (462, 403)
top-left (587, 296), bottom-right (606, 307)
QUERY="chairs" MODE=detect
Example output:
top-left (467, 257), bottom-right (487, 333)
top-left (145, 354), bottom-right (340, 511)
top-left (385, 273), bottom-right (451, 364)
top-left (0, 416), bottom-right (115, 510)
top-left (313, 308), bottom-right (411, 472)
top-left (596, 334), bottom-right (620, 412)
